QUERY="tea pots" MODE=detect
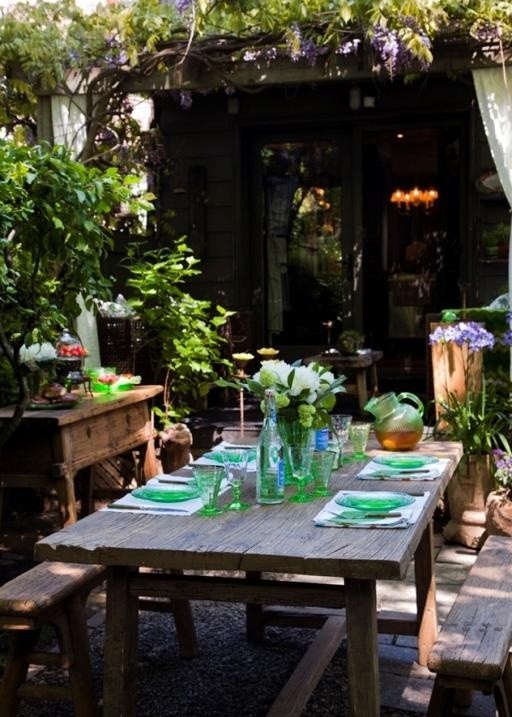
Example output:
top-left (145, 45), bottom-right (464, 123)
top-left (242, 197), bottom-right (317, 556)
top-left (363, 391), bottom-right (425, 449)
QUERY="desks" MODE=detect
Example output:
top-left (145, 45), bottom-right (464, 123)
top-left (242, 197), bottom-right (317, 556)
top-left (1, 381), bottom-right (166, 531)
top-left (302, 345), bottom-right (387, 419)
top-left (28, 412), bottom-right (470, 716)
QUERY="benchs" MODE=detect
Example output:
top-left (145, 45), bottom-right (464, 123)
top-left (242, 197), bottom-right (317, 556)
top-left (1, 552), bottom-right (204, 716)
top-left (418, 530), bottom-right (511, 717)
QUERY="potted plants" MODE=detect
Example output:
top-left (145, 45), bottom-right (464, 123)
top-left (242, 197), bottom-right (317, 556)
top-left (426, 364), bottom-right (512, 524)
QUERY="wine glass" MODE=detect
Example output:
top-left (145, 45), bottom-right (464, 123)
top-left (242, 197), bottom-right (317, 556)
top-left (222, 449), bottom-right (250, 510)
top-left (287, 415), bottom-right (370, 503)
top-left (86, 365), bottom-right (143, 397)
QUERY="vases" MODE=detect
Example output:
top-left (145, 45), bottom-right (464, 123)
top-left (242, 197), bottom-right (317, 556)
top-left (274, 412), bottom-right (320, 486)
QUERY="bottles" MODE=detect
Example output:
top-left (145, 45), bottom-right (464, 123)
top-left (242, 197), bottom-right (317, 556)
top-left (256, 389), bottom-right (285, 504)
top-left (64, 377), bottom-right (72, 393)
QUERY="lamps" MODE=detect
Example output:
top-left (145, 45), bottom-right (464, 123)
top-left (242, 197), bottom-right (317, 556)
top-left (390, 184), bottom-right (438, 215)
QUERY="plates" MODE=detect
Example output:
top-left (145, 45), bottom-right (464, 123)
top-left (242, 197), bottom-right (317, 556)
top-left (373, 452), bottom-right (438, 469)
top-left (132, 485), bottom-right (199, 503)
top-left (29, 401), bottom-right (83, 409)
top-left (202, 450), bottom-right (256, 462)
top-left (334, 490), bottom-right (415, 515)
top-left (34, 358), bottom-right (81, 377)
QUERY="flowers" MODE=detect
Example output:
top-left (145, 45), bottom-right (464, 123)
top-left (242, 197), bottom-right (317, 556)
top-left (233, 356), bottom-right (349, 471)
top-left (427, 319), bottom-right (498, 355)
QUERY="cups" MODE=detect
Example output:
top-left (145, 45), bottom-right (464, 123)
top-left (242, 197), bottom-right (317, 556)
top-left (191, 466), bottom-right (226, 514)
